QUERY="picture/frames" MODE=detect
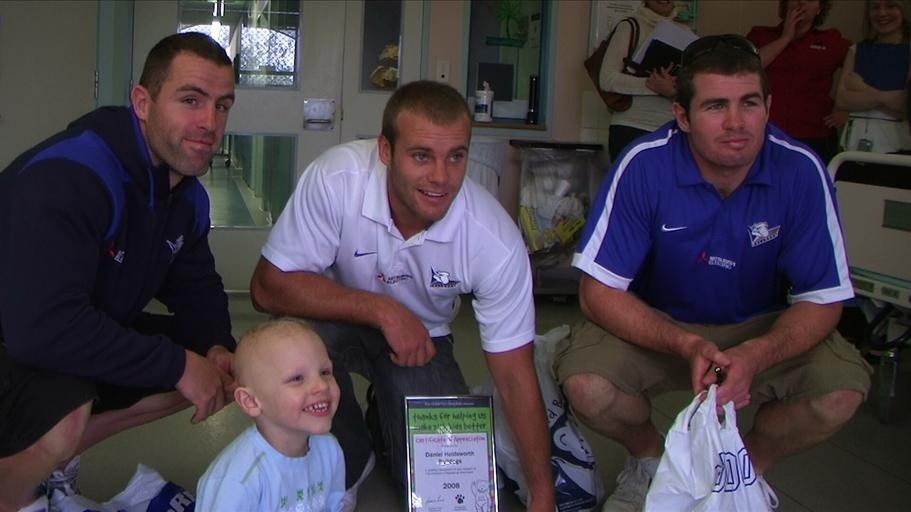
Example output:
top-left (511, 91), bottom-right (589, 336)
top-left (401, 392), bottom-right (498, 512)
top-left (587, 0), bottom-right (698, 65)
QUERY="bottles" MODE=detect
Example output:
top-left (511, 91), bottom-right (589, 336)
top-left (474, 90), bottom-right (496, 124)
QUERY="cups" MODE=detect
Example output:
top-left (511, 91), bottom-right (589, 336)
top-left (555, 180), bottom-right (573, 198)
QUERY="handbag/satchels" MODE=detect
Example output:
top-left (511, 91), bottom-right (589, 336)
top-left (583, 14), bottom-right (641, 115)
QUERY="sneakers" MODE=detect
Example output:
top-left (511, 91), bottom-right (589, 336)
top-left (600, 431), bottom-right (667, 512)
top-left (343, 447), bottom-right (377, 512)
top-left (46, 452), bottom-right (87, 511)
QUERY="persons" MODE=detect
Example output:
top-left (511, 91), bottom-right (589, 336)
top-left (1, 31), bottom-right (242, 510)
top-left (249, 81), bottom-right (554, 512)
top-left (190, 317), bottom-right (348, 510)
top-left (557, 35), bottom-right (875, 511)
top-left (836, 1), bottom-right (911, 155)
top-left (751, 2), bottom-right (851, 163)
top-left (600, 0), bottom-right (703, 162)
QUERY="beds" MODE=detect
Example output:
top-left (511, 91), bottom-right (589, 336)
top-left (821, 149), bottom-right (911, 425)
